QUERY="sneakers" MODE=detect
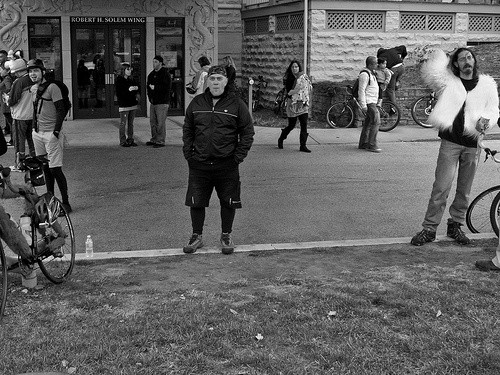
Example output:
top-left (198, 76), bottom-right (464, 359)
top-left (183, 233), bottom-right (204, 253)
top-left (411, 229), bottom-right (436, 245)
top-left (221, 233), bottom-right (234, 254)
top-left (447, 223), bottom-right (470, 245)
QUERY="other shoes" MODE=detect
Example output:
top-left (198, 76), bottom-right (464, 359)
top-left (395, 85), bottom-right (402, 90)
top-left (475, 260), bottom-right (500, 271)
top-left (146, 142), bottom-right (165, 148)
top-left (120, 142), bottom-right (138, 147)
top-left (377, 99), bottom-right (383, 107)
top-left (6, 142), bottom-right (14, 149)
top-left (390, 108), bottom-right (396, 114)
top-left (359, 145), bottom-right (381, 152)
top-left (9, 165), bottom-right (25, 173)
top-left (59, 202), bottom-right (72, 217)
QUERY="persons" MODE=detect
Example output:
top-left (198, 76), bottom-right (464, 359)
top-left (374, 57), bottom-right (391, 108)
top-left (26, 58), bottom-right (72, 217)
top-left (146, 55), bottom-right (171, 148)
top-left (411, 47), bottom-right (499, 246)
top-left (77, 54), bottom-right (105, 108)
top-left (2, 59), bottom-right (35, 172)
top-left (115, 63), bottom-right (141, 148)
top-left (185, 56), bottom-right (212, 98)
top-left (278, 59), bottom-right (312, 153)
top-left (0, 49), bottom-right (28, 198)
top-left (221, 56), bottom-right (236, 95)
top-left (377, 45), bottom-right (407, 116)
top-left (357, 56), bottom-right (381, 153)
top-left (183, 66), bottom-right (256, 255)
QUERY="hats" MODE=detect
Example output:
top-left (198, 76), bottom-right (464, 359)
top-left (10, 58), bottom-right (27, 73)
top-left (208, 65), bottom-right (227, 77)
top-left (27, 58), bottom-right (44, 69)
top-left (154, 55), bottom-right (165, 65)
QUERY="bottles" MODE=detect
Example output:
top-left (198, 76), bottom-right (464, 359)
top-left (86, 235), bottom-right (94, 258)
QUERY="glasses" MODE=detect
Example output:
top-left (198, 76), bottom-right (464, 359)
top-left (127, 67), bottom-right (133, 70)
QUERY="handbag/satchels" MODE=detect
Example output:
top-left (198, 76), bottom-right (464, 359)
top-left (186, 83), bottom-right (197, 94)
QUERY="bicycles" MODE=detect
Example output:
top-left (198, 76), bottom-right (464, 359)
top-left (410, 91), bottom-right (439, 129)
top-left (324, 84), bottom-right (401, 132)
top-left (0, 151), bottom-right (76, 323)
top-left (464, 147), bottom-right (500, 239)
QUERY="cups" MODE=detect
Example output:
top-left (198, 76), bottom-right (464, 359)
top-left (475, 115), bottom-right (489, 132)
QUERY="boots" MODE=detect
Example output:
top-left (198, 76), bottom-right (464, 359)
top-left (300, 132), bottom-right (311, 152)
top-left (278, 129), bottom-right (287, 149)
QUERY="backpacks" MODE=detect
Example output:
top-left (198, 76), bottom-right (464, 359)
top-left (33, 80), bottom-right (71, 138)
top-left (351, 70), bottom-right (370, 99)
top-left (274, 87), bottom-right (287, 119)
